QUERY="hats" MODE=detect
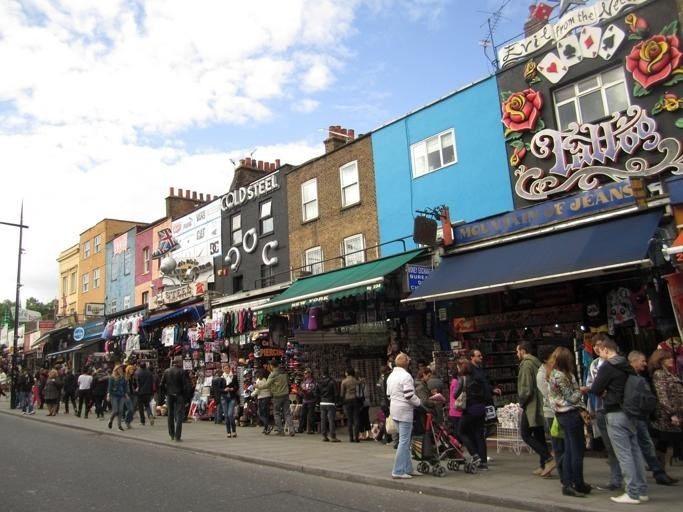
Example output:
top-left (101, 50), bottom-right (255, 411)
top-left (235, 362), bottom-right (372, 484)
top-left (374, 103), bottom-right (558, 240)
top-left (114, 362), bottom-right (126, 370)
top-left (267, 359), bottom-right (279, 365)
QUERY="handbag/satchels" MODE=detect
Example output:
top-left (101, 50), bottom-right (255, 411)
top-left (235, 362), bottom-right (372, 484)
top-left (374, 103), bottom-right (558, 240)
top-left (580, 410), bottom-right (592, 424)
top-left (551, 417), bottom-right (565, 439)
top-left (454, 391), bottom-right (466, 410)
top-left (385, 415), bottom-right (398, 434)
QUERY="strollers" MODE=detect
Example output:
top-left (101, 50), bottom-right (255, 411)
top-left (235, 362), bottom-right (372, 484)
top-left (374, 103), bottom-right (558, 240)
top-left (415, 393), bottom-right (480, 477)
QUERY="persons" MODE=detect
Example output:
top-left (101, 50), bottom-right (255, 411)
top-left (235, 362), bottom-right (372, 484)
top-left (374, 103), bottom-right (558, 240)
top-left (212, 360), bottom-right (295, 438)
top-left (299, 367), bottom-right (364, 442)
top-left (160, 356), bottom-right (193, 442)
top-left (517, 335), bottom-right (682, 503)
top-left (1, 359), bottom-right (159, 431)
top-left (376, 349), bottom-right (501, 479)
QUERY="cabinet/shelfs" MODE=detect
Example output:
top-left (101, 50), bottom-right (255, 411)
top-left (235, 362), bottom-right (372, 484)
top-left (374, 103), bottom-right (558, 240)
top-left (478, 339), bottom-right (544, 410)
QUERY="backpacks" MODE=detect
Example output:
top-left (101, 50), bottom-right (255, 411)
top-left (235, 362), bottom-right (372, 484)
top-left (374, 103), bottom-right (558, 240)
top-left (607, 367), bottom-right (656, 419)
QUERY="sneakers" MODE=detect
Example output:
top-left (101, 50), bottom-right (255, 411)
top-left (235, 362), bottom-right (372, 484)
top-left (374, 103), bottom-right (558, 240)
top-left (477, 465), bottom-right (488, 470)
top-left (472, 455), bottom-right (481, 463)
top-left (532, 462), bottom-right (680, 502)
top-left (392, 473), bottom-right (412, 478)
top-left (409, 470), bottom-right (423, 475)
